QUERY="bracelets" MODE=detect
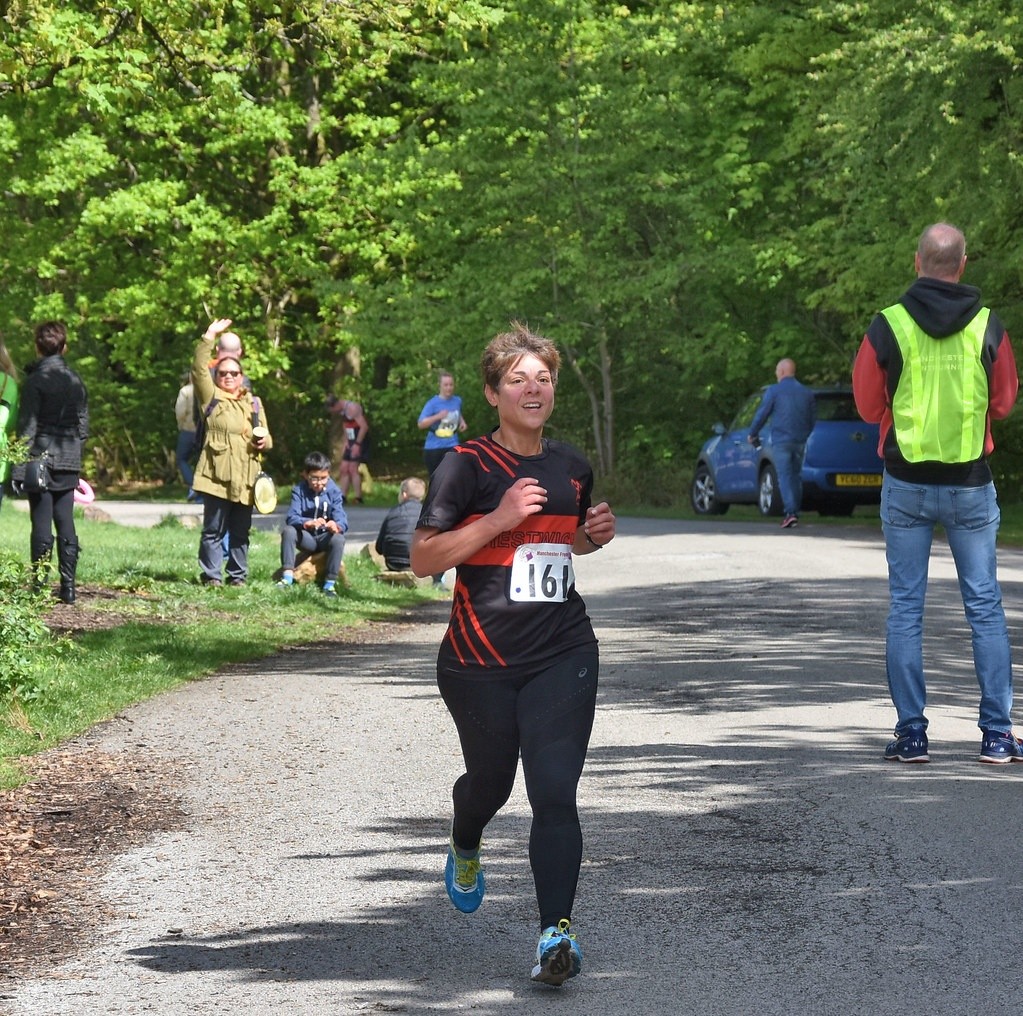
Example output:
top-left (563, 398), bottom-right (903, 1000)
top-left (586, 533), bottom-right (603, 550)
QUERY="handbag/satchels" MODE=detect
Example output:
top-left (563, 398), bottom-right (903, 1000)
top-left (24, 457), bottom-right (49, 493)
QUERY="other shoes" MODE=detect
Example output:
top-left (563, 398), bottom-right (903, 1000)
top-left (433, 582), bottom-right (450, 591)
top-left (185, 485), bottom-right (204, 503)
top-left (323, 579), bottom-right (337, 595)
top-left (277, 575), bottom-right (294, 586)
top-left (780, 511), bottom-right (801, 529)
top-left (205, 579), bottom-right (243, 590)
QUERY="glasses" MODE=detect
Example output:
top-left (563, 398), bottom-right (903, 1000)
top-left (218, 370), bottom-right (241, 377)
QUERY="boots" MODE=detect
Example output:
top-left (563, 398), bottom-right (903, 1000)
top-left (30, 532), bottom-right (55, 595)
top-left (55, 534), bottom-right (82, 604)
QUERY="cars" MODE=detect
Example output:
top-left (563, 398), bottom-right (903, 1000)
top-left (691, 381), bottom-right (884, 515)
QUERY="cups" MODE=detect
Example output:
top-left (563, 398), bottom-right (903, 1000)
top-left (251, 427), bottom-right (269, 448)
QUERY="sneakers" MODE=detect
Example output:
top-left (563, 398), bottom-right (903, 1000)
top-left (531, 917), bottom-right (583, 987)
top-left (980, 730), bottom-right (1023, 764)
top-left (444, 814), bottom-right (485, 915)
top-left (883, 718), bottom-right (931, 763)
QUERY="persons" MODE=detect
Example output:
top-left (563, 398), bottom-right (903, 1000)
top-left (275, 449), bottom-right (350, 600)
top-left (323, 394), bottom-right (370, 505)
top-left (10, 320), bottom-right (91, 605)
top-left (416, 371), bottom-right (467, 488)
top-left (174, 317), bottom-right (273, 588)
top-left (0, 329), bottom-right (19, 510)
top-left (747, 358), bottom-right (817, 528)
top-left (410, 317), bottom-right (618, 987)
top-left (375, 475), bottom-right (454, 593)
top-left (850, 221), bottom-right (1023, 764)
